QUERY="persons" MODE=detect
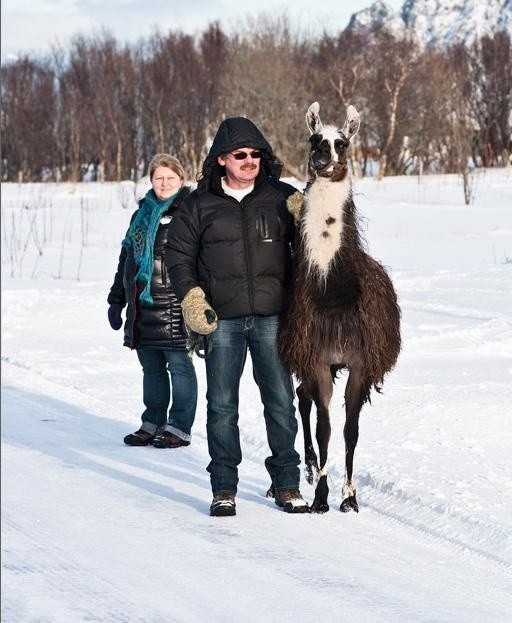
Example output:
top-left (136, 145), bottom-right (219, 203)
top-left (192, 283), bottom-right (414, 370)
top-left (108, 154), bottom-right (199, 448)
top-left (165, 117), bottom-right (309, 515)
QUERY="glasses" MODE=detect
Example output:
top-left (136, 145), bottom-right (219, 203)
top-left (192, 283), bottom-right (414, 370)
top-left (228, 152), bottom-right (263, 160)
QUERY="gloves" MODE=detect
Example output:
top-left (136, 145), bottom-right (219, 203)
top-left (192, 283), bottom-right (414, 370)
top-left (179, 286), bottom-right (218, 336)
top-left (108, 304), bottom-right (123, 330)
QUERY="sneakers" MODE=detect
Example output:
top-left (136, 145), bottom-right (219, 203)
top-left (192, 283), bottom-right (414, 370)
top-left (210, 489), bottom-right (238, 515)
top-left (152, 430), bottom-right (190, 449)
top-left (274, 488), bottom-right (310, 513)
top-left (124, 428), bottom-right (153, 445)
